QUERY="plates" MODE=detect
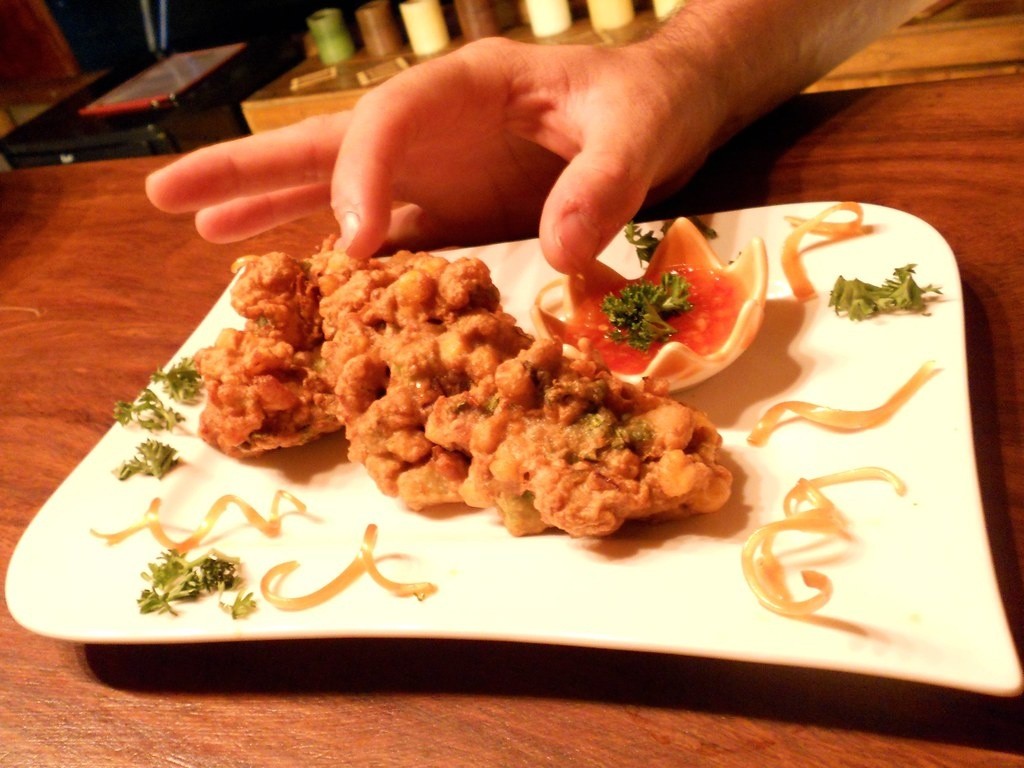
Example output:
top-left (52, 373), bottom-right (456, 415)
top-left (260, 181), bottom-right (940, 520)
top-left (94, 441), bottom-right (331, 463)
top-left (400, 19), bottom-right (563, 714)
top-left (4, 199), bottom-right (1024, 700)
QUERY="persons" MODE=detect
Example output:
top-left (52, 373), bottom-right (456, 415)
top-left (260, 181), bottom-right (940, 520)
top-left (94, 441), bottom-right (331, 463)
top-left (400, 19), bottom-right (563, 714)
top-left (144, 0), bottom-right (939, 273)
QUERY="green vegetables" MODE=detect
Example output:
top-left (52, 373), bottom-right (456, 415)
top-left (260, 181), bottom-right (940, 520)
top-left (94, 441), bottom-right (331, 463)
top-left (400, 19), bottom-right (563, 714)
top-left (597, 212), bottom-right (944, 352)
top-left (113, 356), bottom-right (258, 619)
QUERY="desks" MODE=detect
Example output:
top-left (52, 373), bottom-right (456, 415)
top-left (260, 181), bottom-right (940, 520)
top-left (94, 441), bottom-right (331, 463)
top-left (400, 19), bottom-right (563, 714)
top-left (1, 64), bottom-right (1024, 768)
